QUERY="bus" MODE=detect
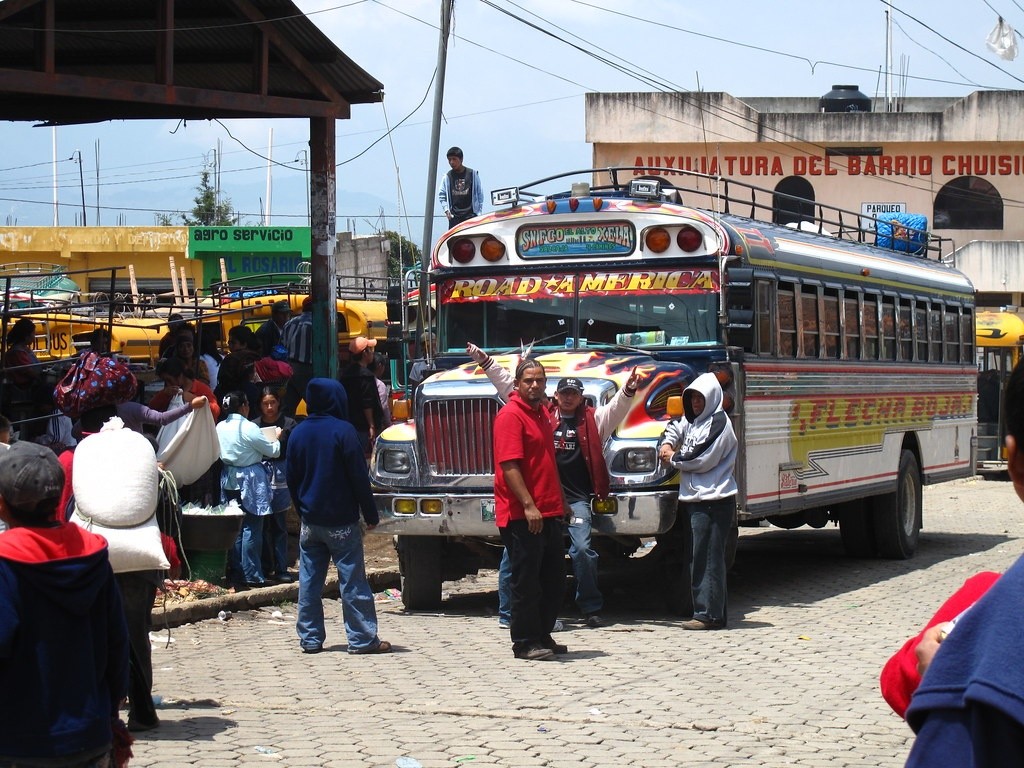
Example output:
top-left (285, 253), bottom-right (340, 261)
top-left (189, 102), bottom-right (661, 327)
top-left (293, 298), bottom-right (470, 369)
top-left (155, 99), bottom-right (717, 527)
top-left (976, 311), bottom-right (1023, 474)
top-left (369, 167), bottom-right (978, 609)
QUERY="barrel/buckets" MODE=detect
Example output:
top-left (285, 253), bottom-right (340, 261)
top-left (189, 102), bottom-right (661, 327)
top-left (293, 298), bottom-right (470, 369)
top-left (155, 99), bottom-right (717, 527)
top-left (184, 550), bottom-right (228, 586)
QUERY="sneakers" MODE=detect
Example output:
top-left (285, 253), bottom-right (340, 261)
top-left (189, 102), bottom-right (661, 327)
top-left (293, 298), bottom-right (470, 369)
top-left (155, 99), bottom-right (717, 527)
top-left (512, 641), bottom-right (554, 659)
top-left (537, 635), bottom-right (567, 654)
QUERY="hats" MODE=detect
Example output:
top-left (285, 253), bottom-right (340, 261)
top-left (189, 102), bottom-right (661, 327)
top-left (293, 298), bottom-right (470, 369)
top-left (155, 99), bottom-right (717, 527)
top-left (176, 330), bottom-right (193, 345)
top-left (272, 301), bottom-right (294, 313)
top-left (349, 337), bottom-right (377, 353)
top-left (557, 377), bottom-right (583, 396)
top-left (302, 297), bottom-right (312, 312)
top-left (0, 441), bottom-right (65, 525)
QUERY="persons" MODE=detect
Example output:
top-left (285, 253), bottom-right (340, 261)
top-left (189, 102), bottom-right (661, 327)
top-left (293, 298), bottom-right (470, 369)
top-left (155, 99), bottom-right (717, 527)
top-left (0, 439), bottom-right (137, 768)
top-left (0, 296), bottom-right (436, 591)
top-left (465, 340), bottom-right (643, 632)
top-left (437, 147), bottom-right (484, 232)
top-left (490, 359), bottom-right (571, 663)
top-left (881, 356), bottom-right (1024, 768)
top-left (654, 372), bottom-right (740, 634)
top-left (53, 430), bottom-right (181, 732)
top-left (284, 377), bottom-right (393, 654)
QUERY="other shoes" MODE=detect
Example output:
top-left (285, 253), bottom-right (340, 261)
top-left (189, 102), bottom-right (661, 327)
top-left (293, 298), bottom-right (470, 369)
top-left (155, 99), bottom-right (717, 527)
top-left (249, 578), bottom-right (272, 586)
top-left (681, 618), bottom-right (722, 630)
top-left (499, 622), bottom-right (510, 628)
top-left (584, 610), bottom-right (602, 627)
top-left (362, 641), bottom-right (392, 653)
top-left (128, 718), bottom-right (160, 730)
top-left (305, 644), bottom-right (322, 653)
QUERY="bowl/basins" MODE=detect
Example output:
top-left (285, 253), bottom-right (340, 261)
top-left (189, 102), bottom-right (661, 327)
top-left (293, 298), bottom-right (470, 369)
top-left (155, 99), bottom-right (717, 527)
top-left (175, 514), bottom-right (246, 552)
